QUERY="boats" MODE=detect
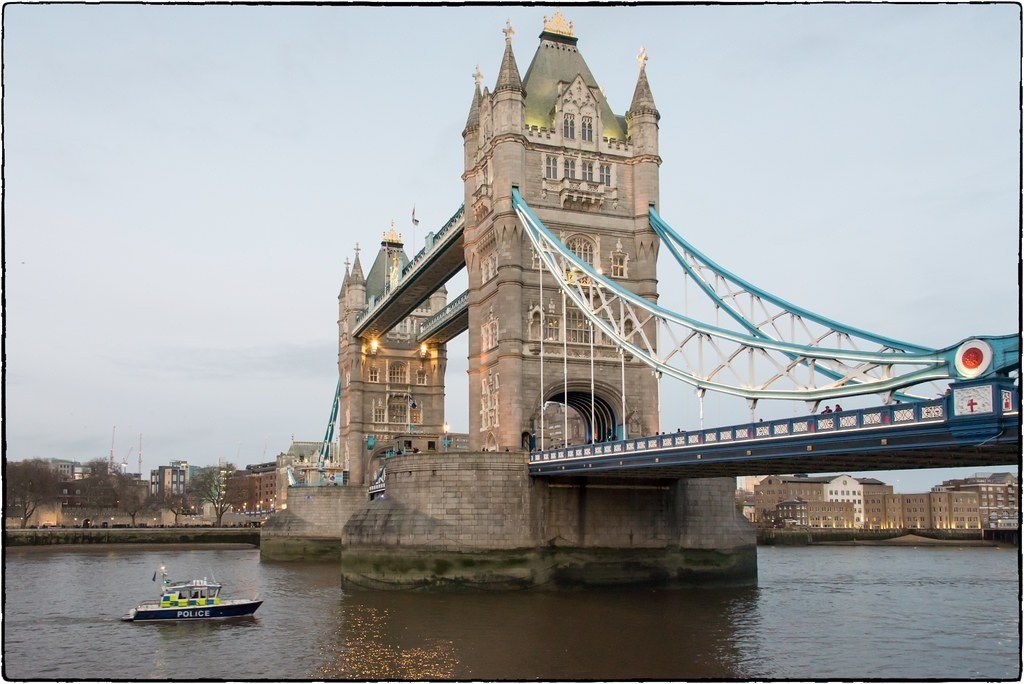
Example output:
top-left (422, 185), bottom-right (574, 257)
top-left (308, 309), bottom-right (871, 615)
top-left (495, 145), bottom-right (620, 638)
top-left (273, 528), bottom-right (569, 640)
top-left (120, 568), bottom-right (265, 623)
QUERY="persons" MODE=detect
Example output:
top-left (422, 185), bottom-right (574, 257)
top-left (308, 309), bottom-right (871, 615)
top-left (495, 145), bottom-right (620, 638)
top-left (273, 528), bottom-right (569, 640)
top-left (395, 448), bottom-right (402, 455)
top-left (531, 445), bottom-right (541, 453)
top-left (834, 404), bottom-right (843, 411)
top-left (821, 406), bottom-right (832, 414)
top-left (413, 447), bottom-right (420, 453)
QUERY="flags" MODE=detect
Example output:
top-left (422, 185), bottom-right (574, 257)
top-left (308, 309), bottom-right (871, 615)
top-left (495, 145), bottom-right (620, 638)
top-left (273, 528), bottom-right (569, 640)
top-left (412, 209), bottom-right (419, 226)
